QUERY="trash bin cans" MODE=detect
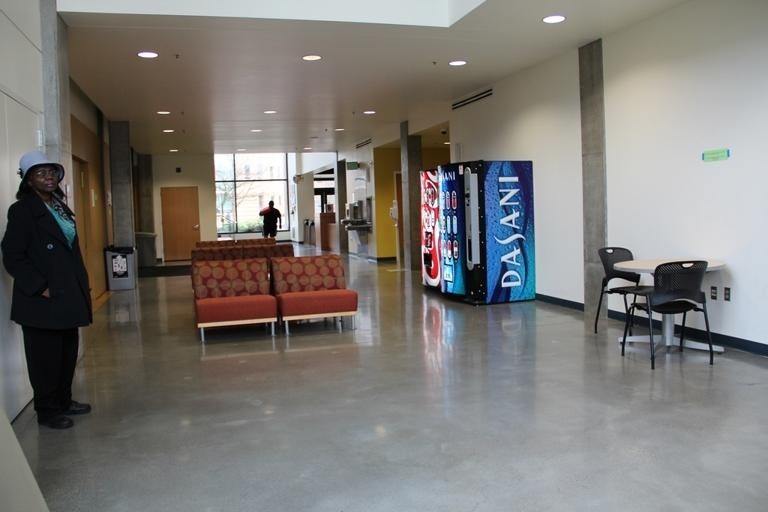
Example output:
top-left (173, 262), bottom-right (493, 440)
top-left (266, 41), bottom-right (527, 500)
top-left (105, 245), bottom-right (135, 291)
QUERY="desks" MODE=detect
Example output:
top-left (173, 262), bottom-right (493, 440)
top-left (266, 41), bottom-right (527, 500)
top-left (613, 260), bottom-right (725, 359)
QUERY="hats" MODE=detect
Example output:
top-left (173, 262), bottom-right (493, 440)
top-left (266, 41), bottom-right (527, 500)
top-left (16, 150), bottom-right (65, 200)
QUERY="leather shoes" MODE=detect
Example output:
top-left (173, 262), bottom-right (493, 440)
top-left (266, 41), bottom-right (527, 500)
top-left (36, 414), bottom-right (74, 430)
top-left (63, 401), bottom-right (91, 414)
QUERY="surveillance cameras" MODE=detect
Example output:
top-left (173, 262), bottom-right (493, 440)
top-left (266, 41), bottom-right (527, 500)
top-left (440, 128), bottom-right (448, 137)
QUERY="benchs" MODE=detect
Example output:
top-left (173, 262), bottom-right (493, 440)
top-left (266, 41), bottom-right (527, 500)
top-left (191, 238), bottom-right (358, 345)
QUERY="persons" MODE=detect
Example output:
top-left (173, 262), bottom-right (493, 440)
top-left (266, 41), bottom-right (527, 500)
top-left (1, 150), bottom-right (94, 428)
top-left (258, 201), bottom-right (282, 238)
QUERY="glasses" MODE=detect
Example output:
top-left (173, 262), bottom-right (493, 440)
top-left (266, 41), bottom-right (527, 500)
top-left (34, 168), bottom-right (57, 178)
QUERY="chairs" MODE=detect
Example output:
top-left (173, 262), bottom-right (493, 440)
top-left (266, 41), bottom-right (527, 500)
top-left (595, 247), bottom-right (654, 343)
top-left (622, 260), bottom-right (713, 370)
